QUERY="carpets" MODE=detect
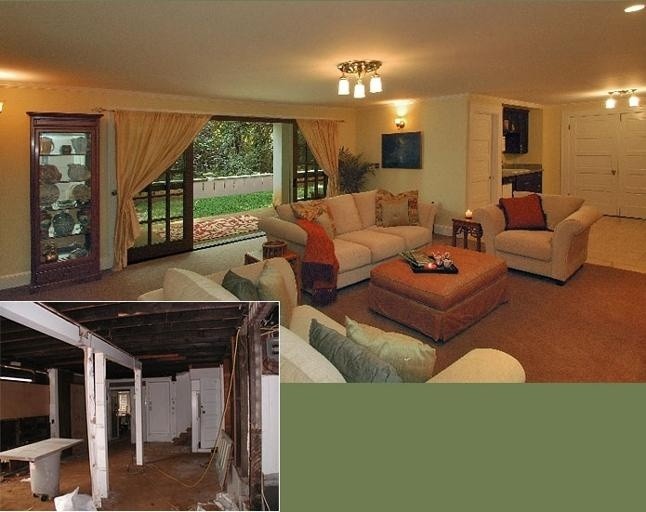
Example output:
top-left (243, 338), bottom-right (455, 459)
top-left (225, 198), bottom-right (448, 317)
top-left (302, 254), bottom-right (646, 383)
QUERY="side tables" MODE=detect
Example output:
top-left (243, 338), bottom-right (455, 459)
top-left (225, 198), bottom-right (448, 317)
top-left (243, 246), bottom-right (297, 269)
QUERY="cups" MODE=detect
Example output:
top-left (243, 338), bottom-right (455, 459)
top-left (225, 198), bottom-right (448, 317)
top-left (63, 145), bottom-right (72, 156)
top-left (503, 119), bottom-right (508, 129)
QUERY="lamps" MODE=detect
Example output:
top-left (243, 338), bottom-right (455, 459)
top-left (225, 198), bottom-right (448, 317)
top-left (335, 58), bottom-right (384, 102)
top-left (605, 89), bottom-right (641, 112)
top-left (394, 118), bottom-right (406, 130)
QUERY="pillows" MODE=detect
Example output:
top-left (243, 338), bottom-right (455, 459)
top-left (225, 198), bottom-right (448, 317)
top-left (343, 312), bottom-right (437, 384)
top-left (499, 194), bottom-right (549, 231)
top-left (379, 200), bottom-right (410, 227)
top-left (373, 188), bottom-right (421, 226)
top-left (254, 261), bottom-right (293, 330)
top-left (313, 212), bottom-right (334, 240)
top-left (293, 202), bottom-right (338, 241)
top-left (217, 269), bottom-right (258, 305)
top-left (304, 313), bottom-right (402, 383)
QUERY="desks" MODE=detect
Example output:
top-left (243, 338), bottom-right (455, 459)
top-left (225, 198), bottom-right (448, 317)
top-left (450, 215), bottom-right (487, 253)
top-left (0, 436), bottom-right (87, 503)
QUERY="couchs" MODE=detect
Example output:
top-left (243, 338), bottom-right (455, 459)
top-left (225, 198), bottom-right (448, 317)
top-left (135, 256), bottom-right (529, 384)
top-left (257, 189), bottom-right (443, 302)
top-left (472, 190), bottom-right (605, 286)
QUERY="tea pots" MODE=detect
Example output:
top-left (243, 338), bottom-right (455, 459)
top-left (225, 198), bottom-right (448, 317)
top-left (431, 249), bottom-right (453, 268)
top-left (38, 136), bottom-right (53, 155)
top-left (70, 137), bottom-right (87, 155)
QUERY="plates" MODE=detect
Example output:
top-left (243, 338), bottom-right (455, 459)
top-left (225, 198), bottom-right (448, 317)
top-left (38, 185), bottom-right (61, 205)
top-left (38, 163), bottom-right (61, 184)
top-left (68, 165), bottom-right (90, 181)
top-left (71, 184), bottom-right (90, 202)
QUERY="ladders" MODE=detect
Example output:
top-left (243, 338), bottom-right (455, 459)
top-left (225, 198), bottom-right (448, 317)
top-left (214, 429), bottom-right (235, 491)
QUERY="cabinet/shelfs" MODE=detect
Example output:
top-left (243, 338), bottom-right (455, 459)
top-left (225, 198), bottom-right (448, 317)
top-left (506, 168), bottom-right (543, 192)
top-left (23, 111), bottom-right (104, 293)
top-left (504, 104), bottom-right (529, 156)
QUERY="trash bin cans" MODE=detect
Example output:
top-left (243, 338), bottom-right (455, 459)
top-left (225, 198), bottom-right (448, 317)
top-left (29, 452), bottom-right (61, 502)
